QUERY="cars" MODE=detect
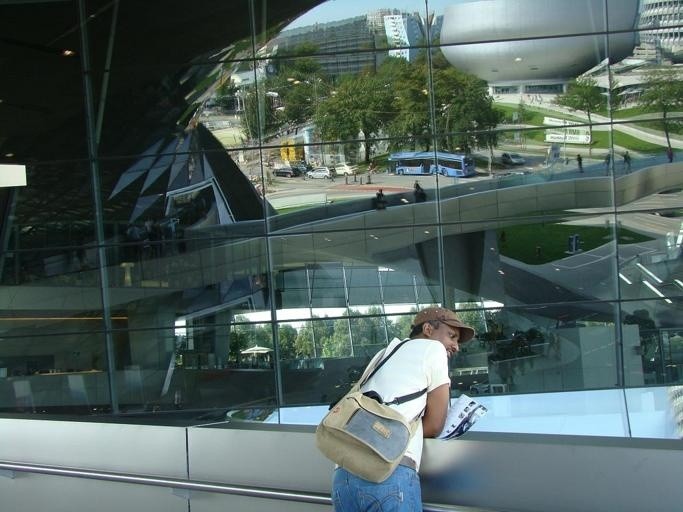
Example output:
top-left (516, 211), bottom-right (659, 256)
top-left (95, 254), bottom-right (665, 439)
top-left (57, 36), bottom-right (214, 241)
top-left (257, 153), bottom-right (336, 180)
top-left (229, 355), bottom-right (339, 374)
top-left (333, 161), bottom-right (360, 177)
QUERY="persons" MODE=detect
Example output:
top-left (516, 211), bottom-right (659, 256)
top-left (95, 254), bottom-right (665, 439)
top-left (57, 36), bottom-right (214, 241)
top-left (577, 154), bottom-right (583, 170)
top-left (331, 306), bottom-right (474, 512)
top-left (376, 189), bottom-right (384, 209)
top-left (125, 217), bottom-right (185, 261)
top-left (415, 185), bottom-right (425, 202)
top-left (667, 148), bottom-right (673, 162)
top-left (623, 151), bottom-right (632, 174)
top-left (604, 154), bottom-right (611, 175)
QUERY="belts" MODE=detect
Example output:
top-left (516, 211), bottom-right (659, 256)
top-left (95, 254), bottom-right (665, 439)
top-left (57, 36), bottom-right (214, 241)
top-left (333, 455), bottom-right (418, 472)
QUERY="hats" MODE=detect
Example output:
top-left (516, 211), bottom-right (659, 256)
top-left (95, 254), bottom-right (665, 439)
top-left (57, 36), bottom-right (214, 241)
top-left (411, 306), bottom-right (475, 344)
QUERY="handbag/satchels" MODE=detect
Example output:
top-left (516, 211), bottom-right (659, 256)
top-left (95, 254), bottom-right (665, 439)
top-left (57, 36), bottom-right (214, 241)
top-left (313, 339), bottom-right (428, 484)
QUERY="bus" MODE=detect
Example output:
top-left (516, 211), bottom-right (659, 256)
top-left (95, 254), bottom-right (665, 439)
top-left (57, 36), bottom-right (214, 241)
top-left (198, 92), bottom-right (281, 119)
top-left (387, 151), bottom-right (475, 177)
top-left (500, 152), bottom-right (526, 166)
